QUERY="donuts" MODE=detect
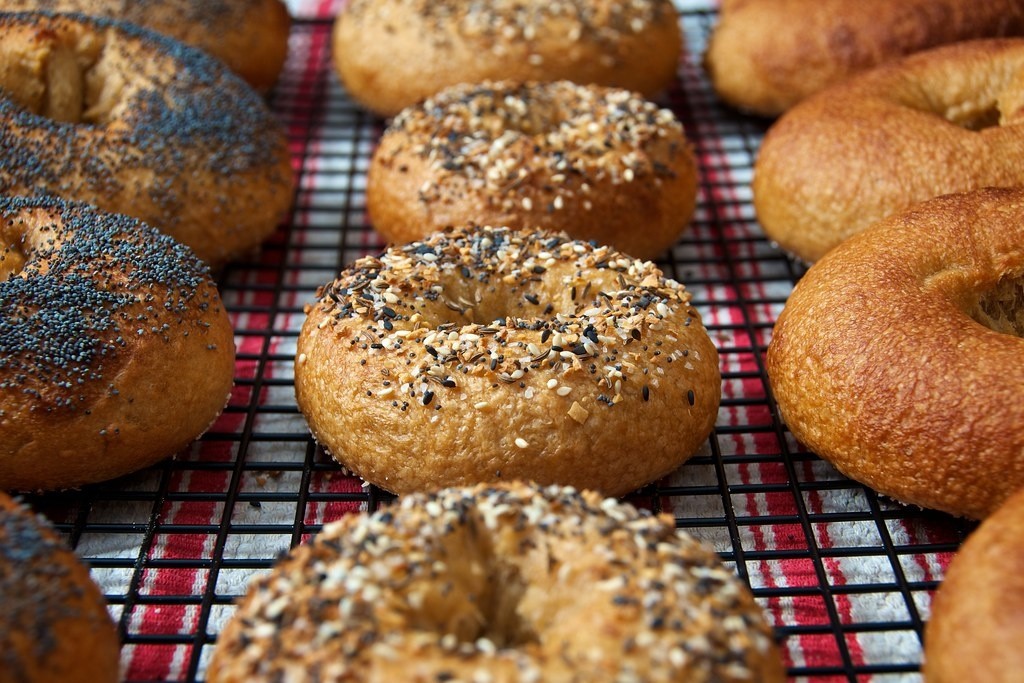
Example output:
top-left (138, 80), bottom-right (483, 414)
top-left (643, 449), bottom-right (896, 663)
top-left (0, 1), bottom-right (1024, 683)
